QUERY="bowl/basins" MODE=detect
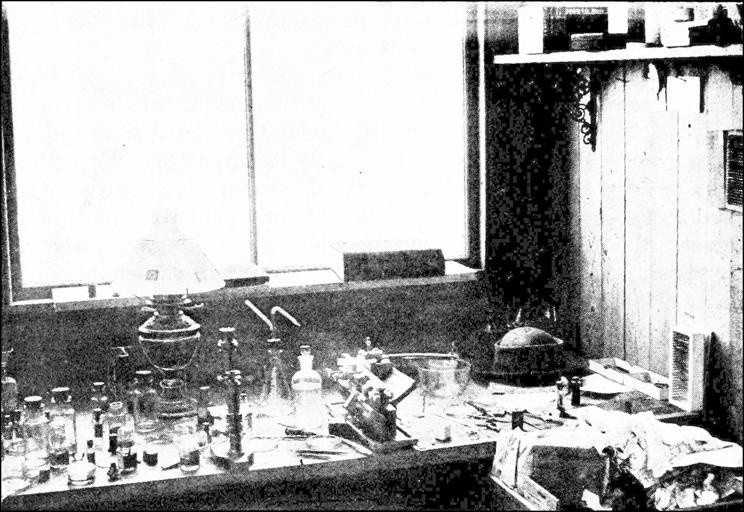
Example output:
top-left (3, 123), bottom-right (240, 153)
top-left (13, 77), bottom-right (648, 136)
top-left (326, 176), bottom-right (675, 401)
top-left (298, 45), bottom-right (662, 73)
top-left (413, 358), bottom-right (472, 398)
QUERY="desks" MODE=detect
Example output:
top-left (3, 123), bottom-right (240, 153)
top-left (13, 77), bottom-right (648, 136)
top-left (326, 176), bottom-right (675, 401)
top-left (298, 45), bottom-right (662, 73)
top-left (0, 366), bottom-right (701, 509)
